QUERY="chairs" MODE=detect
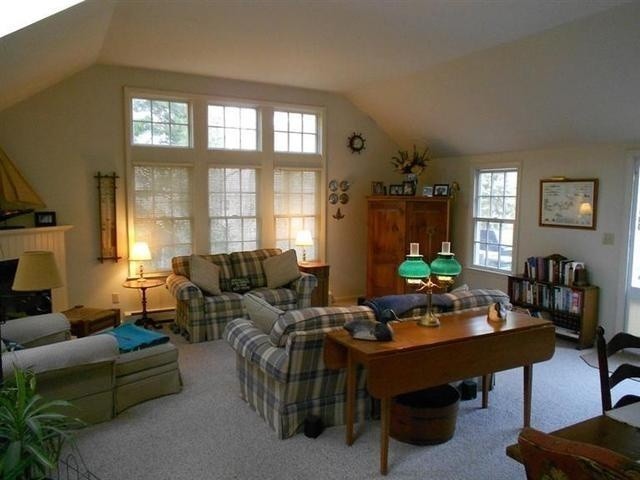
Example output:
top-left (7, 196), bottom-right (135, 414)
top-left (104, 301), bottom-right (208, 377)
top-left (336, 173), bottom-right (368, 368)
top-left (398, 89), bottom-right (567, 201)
top-left (0, 313), bottom-right (182, 429)
top-left (597, 326), bottom-right (640, 414)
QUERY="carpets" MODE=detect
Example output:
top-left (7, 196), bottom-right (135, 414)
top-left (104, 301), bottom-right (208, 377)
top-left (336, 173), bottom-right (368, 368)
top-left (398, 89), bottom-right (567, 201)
top-left (579, 350), bottom-right (640, 383)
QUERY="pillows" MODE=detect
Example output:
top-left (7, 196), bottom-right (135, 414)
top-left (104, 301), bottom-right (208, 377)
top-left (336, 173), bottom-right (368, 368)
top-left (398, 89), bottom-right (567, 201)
top-left (188, 252), bottom-right (221, 298)
top-left (262, 248), bottom-right (301, 288)
top-left (244, 293), bottom-right (284, 331)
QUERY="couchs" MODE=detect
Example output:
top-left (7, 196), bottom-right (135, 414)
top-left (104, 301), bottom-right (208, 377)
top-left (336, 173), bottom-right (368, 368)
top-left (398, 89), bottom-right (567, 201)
top-left (164, 248), bottom-right (318, 344)
top-left (222, 290), bottom-right (510, 439)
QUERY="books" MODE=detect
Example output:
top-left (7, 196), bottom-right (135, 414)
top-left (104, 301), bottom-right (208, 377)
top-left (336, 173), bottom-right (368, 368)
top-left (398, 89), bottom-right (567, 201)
top-left (510, 253), bottom-right (588, 336)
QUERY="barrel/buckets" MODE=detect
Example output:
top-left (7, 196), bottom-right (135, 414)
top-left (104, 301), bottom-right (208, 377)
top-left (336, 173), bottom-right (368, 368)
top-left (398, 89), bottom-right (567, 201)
top-left (388, 383), bottom-right (461, 445)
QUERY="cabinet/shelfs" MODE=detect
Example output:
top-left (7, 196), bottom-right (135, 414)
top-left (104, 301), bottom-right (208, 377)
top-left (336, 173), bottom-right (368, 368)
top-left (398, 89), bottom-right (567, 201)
top-left (366, 196), bottom-right (452, 295)
top-left (507, 276), bottom-right (600, 350)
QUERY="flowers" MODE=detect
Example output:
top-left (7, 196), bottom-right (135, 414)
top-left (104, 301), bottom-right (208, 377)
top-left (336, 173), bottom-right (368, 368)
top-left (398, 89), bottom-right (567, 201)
top-left (390, 144), bottom-right (432, 176)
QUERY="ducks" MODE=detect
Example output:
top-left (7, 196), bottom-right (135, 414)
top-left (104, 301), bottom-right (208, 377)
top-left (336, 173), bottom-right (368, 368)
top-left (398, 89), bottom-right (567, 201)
top-left (343, 308), bottom-right (402, 341)
top-left (488, 302), bottom-right (507, 322)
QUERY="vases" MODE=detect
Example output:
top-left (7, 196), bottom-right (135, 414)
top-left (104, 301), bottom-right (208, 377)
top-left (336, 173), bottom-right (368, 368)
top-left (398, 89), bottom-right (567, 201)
top-left (408, 174), bottom-right (419, 181)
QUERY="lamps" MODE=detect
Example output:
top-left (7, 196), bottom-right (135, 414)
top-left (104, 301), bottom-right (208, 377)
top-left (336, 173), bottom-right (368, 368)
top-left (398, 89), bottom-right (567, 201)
top-left (296, 231), bottom-right (314, 265)
top-left (12, 251), bottom-right (66, 316)
top-left (398, 241), bottom-right (462, 327)
top-left (128, 243), bottom-right (152, 281)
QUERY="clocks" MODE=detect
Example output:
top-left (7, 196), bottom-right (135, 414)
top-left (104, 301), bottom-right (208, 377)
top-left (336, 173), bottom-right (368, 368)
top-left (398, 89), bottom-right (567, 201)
top-left (345, 132), bottom-right (367, 155)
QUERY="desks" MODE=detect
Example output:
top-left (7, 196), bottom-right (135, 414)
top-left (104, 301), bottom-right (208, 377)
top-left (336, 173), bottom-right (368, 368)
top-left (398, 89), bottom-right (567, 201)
top-left (0, 225), bottom-right (74, 314)
top-left (123, 280), bottom-right (165, 327)
top-left (61, 305), bottom-right (121, 338)
top-left (506, 402), bottom-right (640, 480)
top-left (323, 307), bottom-right (556, 475)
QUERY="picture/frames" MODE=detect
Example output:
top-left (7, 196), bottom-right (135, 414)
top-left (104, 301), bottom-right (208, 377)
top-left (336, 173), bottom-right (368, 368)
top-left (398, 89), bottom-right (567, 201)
top-left (34, 212), bottom-right (56, 226)
top-left (422, 184), bottom-right (449, 197)
top-left (371, 182), bottom-right (387, 196)
top-left (389, 181), bottom-right (418, 196)
top-left (538, 176), bottom-right (599, 230)
